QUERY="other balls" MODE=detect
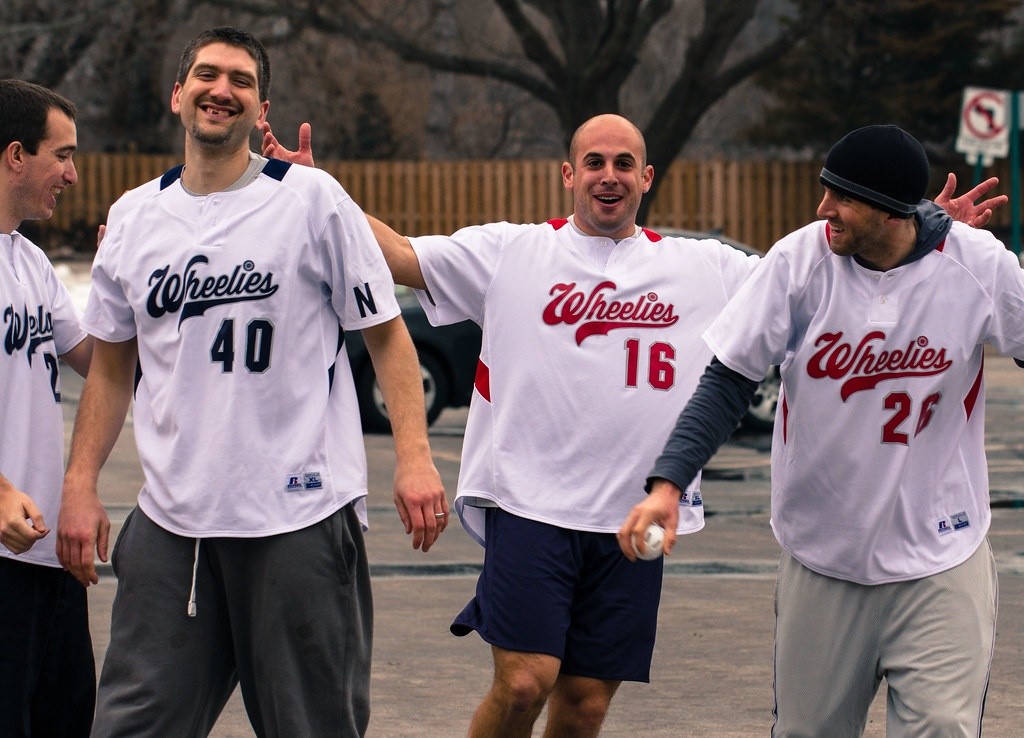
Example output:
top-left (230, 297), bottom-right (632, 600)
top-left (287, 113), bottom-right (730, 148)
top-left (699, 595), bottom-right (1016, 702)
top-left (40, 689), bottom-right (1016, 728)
top-left (631, 523), bottom-right (666, 560)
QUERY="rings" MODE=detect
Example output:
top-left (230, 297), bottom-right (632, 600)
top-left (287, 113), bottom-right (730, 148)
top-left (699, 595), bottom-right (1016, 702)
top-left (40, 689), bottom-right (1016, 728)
top-left (435, 513), bottom-right (444, 519)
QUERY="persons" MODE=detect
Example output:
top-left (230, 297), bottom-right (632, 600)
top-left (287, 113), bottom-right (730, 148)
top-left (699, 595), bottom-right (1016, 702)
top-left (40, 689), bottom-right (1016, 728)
top-left (55, 27), bottom-right (450, 738)
top-left (262, 114), bottom-right (1024, 738)
top-left (0, 79), bottom-right (106, 738)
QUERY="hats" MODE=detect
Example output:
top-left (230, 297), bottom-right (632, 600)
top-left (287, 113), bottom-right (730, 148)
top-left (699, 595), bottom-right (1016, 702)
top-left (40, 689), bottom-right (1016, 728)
top-left (818, 124), bottom-right (929, 217)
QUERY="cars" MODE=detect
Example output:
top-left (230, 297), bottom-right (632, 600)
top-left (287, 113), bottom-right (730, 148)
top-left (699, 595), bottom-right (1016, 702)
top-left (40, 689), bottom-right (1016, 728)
top-left (334, 224), bottom-right (817, 435)
